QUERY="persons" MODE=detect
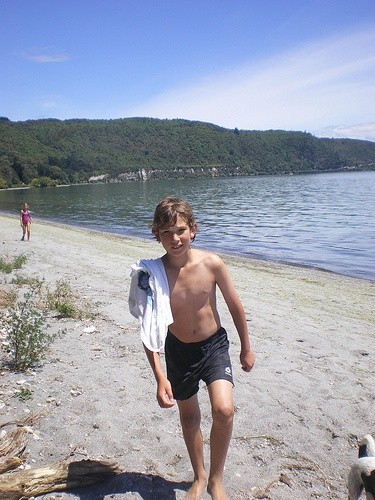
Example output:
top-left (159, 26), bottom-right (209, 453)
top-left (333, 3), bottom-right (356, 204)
top-left (128, 197), bottom-right (256, 500)
top-left (20, 201), bottom-right (33, 241)
